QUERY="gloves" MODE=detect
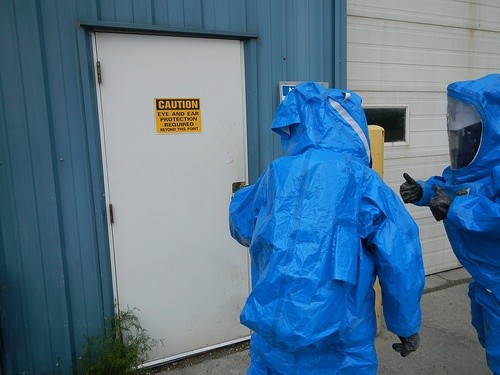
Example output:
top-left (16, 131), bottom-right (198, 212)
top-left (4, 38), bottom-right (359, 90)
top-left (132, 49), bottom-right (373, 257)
top-left (428, 185), bottom-right (451, 221)
top-left (399, 173), bottom-right (423, 204)
top-left (391, 333), bottom-right (421, 358)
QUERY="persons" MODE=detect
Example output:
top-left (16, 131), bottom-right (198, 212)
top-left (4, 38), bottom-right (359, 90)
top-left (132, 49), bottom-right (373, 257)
top-left (399, 73), bottom-right (500, 375)
top-left (229, 81), bottom-right (425, 375)
